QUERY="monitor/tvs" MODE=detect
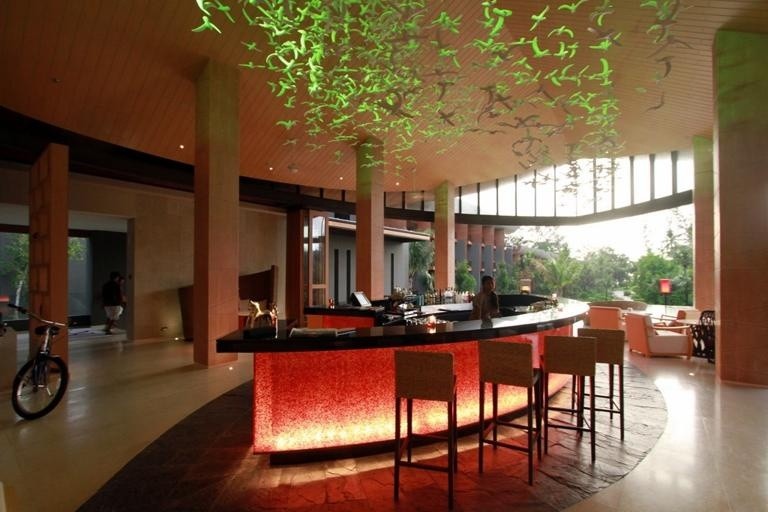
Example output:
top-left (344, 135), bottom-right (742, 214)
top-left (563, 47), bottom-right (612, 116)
top-left (351, 292), bottom-right (372, 307)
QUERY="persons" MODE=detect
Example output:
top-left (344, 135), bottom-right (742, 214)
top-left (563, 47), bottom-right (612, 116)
top-left (469, 275), bottom-right (501, 320)
top-left (411, 266), bottom-right (436, 295)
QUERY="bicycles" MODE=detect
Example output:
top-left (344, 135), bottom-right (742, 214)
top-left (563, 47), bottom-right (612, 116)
top-left (8, 304), bottom-right (67, 420)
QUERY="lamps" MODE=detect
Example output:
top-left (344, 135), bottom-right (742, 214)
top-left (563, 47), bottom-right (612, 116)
top-left (660, 279), bottom-right (672, 315)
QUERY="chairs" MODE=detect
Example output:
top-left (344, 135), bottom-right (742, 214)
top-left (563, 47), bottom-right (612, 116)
top-left (540, 334), bottom-right (596, 466)
top-left (590, 306), bottom-right (693, 360)
top-left (476, 338), bottom-right (542, 486)
top-left (572, 329), bottom-right (624, 439)
top-left (688, 311), bottom-right (716, 364)
top-left (395, 348), bottom-right (458, 509)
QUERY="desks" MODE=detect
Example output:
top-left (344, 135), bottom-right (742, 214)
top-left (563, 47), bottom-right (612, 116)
top-left (676, 319), bottom-right (715, 358)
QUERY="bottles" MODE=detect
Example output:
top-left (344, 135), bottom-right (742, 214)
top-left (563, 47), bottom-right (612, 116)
top-left (425, 286), bottom-right (475, 300)
top-left (390, 286), bottom-right (417, 294)
